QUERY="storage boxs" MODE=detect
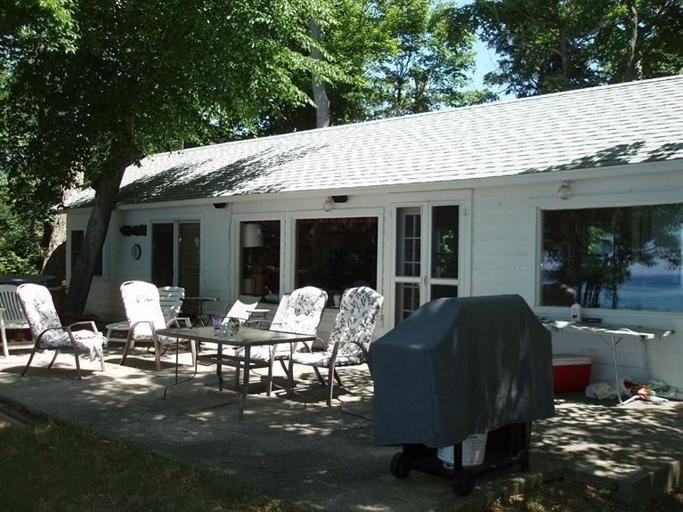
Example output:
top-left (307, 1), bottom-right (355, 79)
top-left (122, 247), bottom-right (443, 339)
top-left (551, 354), bottom-right (592, 395)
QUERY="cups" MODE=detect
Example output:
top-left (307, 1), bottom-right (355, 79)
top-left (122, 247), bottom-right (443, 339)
top-left (212, 317), bottom-right (222, 329)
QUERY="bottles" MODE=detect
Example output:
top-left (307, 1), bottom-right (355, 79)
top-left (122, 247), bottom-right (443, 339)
top-left (227, 317), bottom-right (239, 336)
top-left (570, 299), bottom-right (582, 324)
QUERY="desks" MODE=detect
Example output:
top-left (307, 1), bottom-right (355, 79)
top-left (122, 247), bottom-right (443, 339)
top-left (155, 325), bottom-right (317, 422)
top-left (537, 318), bottom-right (674, 406)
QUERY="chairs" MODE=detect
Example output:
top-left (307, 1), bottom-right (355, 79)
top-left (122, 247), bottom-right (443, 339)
top-left (16, 283), bottom-right (109, 381)
top-left (119, 280), bottom-right (197, 372)
top-left (0, 284), bottom-right (49, 357)
top-left (235, 287), bottom-right (329, 398)
top-left (198, 295), bottom-right (262, 365)
top-left (276, 286), bottom-right (384, 410)
top-left (103, 286), bottom-right (192, 348)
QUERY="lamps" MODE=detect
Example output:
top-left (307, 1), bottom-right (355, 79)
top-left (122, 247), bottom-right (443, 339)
top-left (557, 178), bottom-right (573, 200)
top-left (322, 195), bottom-right (334, 213)
top-left (240, 223), bottom-right (264, 273)
